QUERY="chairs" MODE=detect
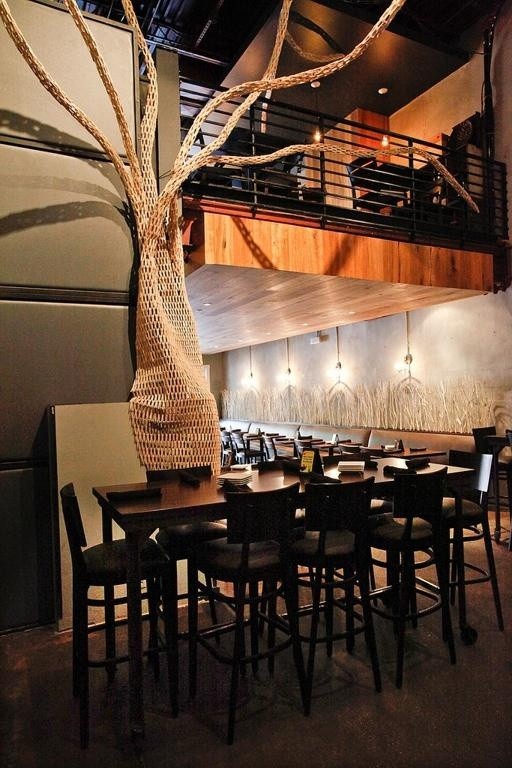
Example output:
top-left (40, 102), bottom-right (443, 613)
top-left (345, 156), bottom-right (389, 214)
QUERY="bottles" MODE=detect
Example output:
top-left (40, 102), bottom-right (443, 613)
top-left (393, 439), bottom-right (405, 453)
top-left (254, 427), bottom-right (260, 436)
top-left (330, 433), bottom-right (339, 445)
top-left (294, 431), bottom-right (301, 439)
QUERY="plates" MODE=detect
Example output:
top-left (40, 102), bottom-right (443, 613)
top-left (337, 460), bottom-right (366, 473)
top-left (383, 449), bottom-right (402, 454)
top-left (216, 472), bottom-right (253, 488)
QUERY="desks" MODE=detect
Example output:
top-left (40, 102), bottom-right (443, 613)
top-left (378, 161), bottom-right (437, 224)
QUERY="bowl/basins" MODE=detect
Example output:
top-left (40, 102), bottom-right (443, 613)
top-left (384, 444), bottom-right (395, 451)
